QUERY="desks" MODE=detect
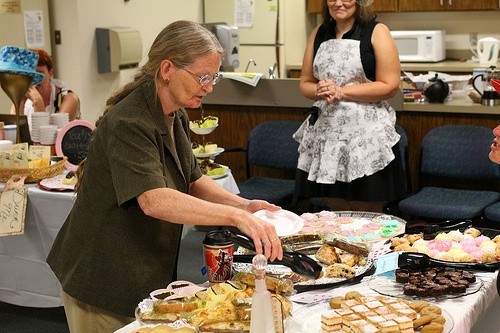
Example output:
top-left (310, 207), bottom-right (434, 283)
top-left (0, 172), bottom-right (241, 308)
top-left (111, 269), bottom-right (500, 333)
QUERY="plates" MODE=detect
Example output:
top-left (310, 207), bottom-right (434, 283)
top-left (284, 295), bottom-right (455, 333)
top-left (367, 269), bottom-right (483, 299)
top-left (312, 211), bottom-right (407, 242)
top-left (37, 178), bottom-right (75, 192)
top-left (29, 111), bottom-right (70, 145)
top-left (426, 227), bottom-right (500, 269)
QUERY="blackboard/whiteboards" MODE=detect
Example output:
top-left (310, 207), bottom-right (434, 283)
top-left (55, 120), bottom-right (96, 172)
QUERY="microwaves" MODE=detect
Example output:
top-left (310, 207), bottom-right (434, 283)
top-left (388, 31), bottom-right (447, 63)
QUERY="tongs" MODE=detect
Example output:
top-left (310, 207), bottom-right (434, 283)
top-left (397, 250), bottom-right (500, 274)
top-left (222, 230), bottom-right (322, 280)
top-left (423, 218), bottom-right (472, 237)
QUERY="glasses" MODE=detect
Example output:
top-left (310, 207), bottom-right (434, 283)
top-left (181, 67), bottom-right (222, 85)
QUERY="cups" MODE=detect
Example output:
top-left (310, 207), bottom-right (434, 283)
top-left (4, 125), bottom-right (17, 145)
top-left (203, 229), bottom-right (235, 283)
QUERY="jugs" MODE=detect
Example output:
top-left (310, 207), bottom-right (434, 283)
top-left (476, 37), bottom-right (500, 68)
top-left (472, 65), bottom-right (500, 106)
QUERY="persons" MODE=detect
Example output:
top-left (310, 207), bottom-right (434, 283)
top-left (489, 125), bottom-right (500, 165)
top-left (10, 49), bottom-right (83, 122)
top-left (45, 20), bottom-right (283, 333)
top-left (291, 0), bottom-right (401, 203)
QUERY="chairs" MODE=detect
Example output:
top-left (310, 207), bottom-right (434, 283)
top-left (223, 121), bottom-right (500, 229)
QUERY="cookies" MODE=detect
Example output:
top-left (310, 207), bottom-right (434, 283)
top-left (301, 210), bottom-right (404, 244)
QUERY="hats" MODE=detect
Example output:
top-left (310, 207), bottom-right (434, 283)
top-left (0, 45), bottom-right (45, 86)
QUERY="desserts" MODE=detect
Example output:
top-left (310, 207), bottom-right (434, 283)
top-left (189, 116), bottom-right (225, 176)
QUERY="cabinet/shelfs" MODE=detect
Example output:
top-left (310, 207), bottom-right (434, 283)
top-left (306, 0), bottom-right (500, 14)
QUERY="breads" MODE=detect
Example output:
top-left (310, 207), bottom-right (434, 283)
top-left (125, 228), bottom-right (500, 333)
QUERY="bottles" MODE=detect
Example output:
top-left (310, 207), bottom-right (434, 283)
top-left (249, 254), bottom-right (276, 333)
top-left (423, 73), bottom-right (449, 103)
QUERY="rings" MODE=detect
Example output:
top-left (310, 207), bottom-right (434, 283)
top-left (327, 87), bottom-right (328, 90)
top-left (270, 238), bottom-right (277, 242)
